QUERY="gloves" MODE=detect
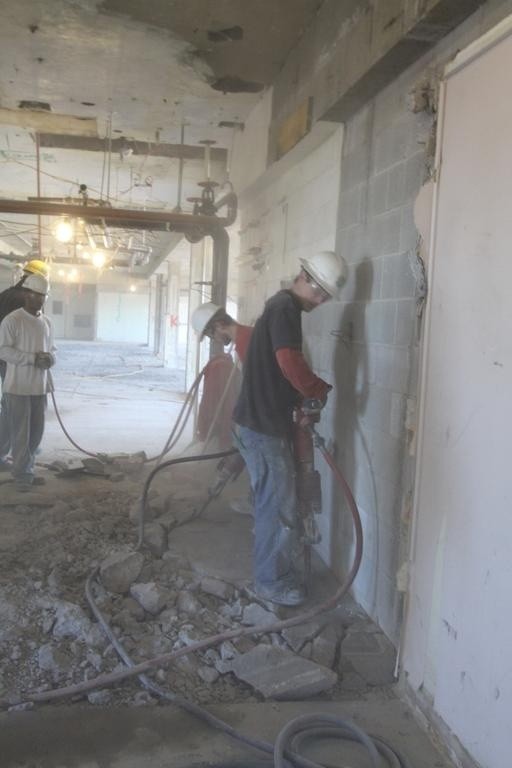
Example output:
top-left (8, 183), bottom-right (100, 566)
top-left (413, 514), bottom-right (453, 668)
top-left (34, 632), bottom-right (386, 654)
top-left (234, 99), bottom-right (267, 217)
top-left (35, 351), bottom-right (54, 368)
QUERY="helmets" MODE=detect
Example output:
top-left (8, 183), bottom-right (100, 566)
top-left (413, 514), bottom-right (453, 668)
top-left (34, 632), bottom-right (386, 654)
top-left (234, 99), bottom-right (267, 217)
top-left (21, 260), bottom-right (49, 295)
top-left (295, 250), bottom-right (349, 302)
top-left (190, 303), bottom-right (223, 344)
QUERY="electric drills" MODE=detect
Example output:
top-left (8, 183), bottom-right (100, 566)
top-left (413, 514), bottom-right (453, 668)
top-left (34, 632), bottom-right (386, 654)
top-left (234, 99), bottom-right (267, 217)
top-left (289, 374), bottom-right (333, 580)
top-left (194, 447), bottom-right (246, 519)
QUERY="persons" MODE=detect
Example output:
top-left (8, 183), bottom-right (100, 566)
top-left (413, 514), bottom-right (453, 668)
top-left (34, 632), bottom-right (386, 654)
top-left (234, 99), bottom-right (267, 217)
top-left (0, 260), bottom-right (58, 493)
top-left (193, 303), bottom-right (254, 363)
top-left (231, 251), bottom-right (348, 606)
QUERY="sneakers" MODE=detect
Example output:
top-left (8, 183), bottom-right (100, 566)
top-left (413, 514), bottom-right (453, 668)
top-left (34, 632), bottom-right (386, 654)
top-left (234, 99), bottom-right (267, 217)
top-left (0, 456), bottom-right (47, 492)
top-left (254, 580), bottom-right (306, 607)
